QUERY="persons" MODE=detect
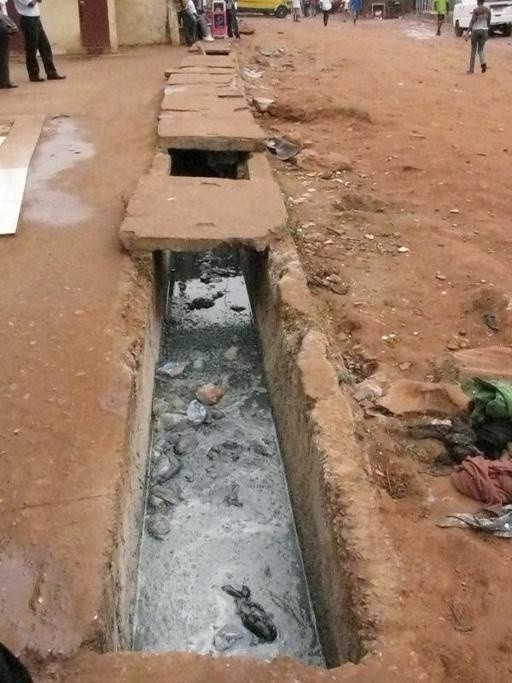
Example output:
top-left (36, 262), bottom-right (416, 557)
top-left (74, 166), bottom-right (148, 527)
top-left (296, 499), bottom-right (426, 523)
top-left (433, 0), bottom-right (449, 35)
top-left (224, 0), bottom-right (240, 38)
top-left (462, 0), bottom-right (491, 74)
top-left (175, 0), bottom-right (216, 46)
top-left (0, 0), bottom-right (20, 88)
top-left (13, 0), bottom-right (66, 81)
top-left (291, 0), bottom-right (363, 26)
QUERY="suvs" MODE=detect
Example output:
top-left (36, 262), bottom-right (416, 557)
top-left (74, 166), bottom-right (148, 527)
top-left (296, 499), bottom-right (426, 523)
top-left (452, 0), bottom-right (512, 38)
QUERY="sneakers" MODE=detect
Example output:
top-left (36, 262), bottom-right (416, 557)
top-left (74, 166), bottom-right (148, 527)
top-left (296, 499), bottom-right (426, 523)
top-left (0, 83), bottom-right (19, 88)
top-left (29, 78), bottom-right (45, 82)
top-left (47, 75), bottom-right (65, 79)
top-left (481, 63), bottom-right (486, 72)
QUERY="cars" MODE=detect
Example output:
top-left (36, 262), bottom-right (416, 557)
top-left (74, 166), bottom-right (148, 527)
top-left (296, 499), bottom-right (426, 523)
top-left (234, 0), bottom-right (293, 18)
